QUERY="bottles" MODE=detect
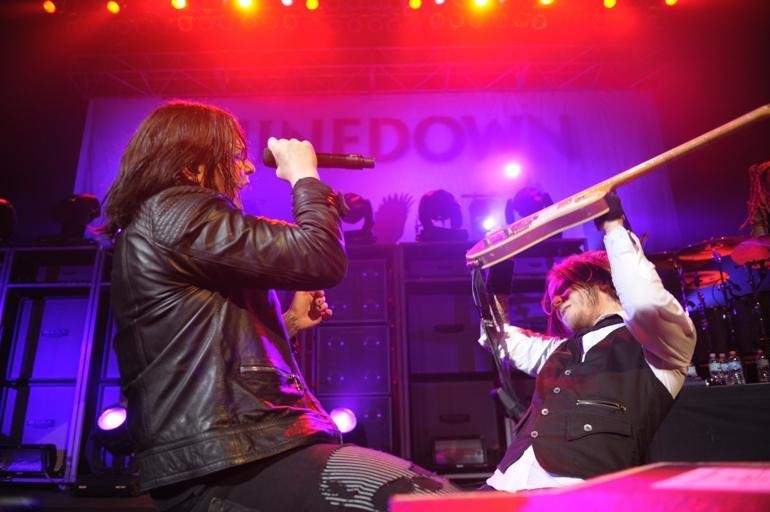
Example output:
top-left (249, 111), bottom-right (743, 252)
top-left (754, 349), bottom-right (770, 382)
top-left (709, 350), bottom-right (745, 385)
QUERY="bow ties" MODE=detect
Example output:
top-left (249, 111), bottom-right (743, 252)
top-left (576, 315), bottom-right (624, 337)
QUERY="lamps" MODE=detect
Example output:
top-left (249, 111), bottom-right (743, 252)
top-left (505, 186), bottom-right (563, 238)
top-left (342, 194), bottom-right (376, 246)
top-left (415, 190), bottom-right (469, 241)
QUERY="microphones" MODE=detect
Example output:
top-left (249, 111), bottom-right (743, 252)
top-left (261, 147), bottom-right (377, 170)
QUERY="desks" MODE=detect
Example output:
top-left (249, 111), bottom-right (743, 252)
top-left (645, 382), bottom-right (770, 462)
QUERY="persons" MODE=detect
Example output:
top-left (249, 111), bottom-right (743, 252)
top-left (453, 180), bottom-right (702, 495)
top-left (85, 96), bottom-right (465, 512)
top-left (689, 314), bottom-right (728, 375)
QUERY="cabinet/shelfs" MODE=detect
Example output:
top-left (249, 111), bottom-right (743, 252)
top-left (0, 231), bottom-right (597, 512)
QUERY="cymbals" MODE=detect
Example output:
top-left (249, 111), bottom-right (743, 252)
top-left (673, 237), bottom-right (750, 261)
top-left (672, 270), bottom-right (730, 290)
top-left (646, 250), bottom-right (705, 270)
top-left (731, 236), bottom-right (770, 269)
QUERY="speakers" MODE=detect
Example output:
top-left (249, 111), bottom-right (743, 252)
top-left (407, 285), bottom-right (547, 464)
top-left (1, 284), bottom-right (130, 477)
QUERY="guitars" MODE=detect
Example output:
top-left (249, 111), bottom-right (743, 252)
top-left (466, 105), bottom-right (770, 271)
